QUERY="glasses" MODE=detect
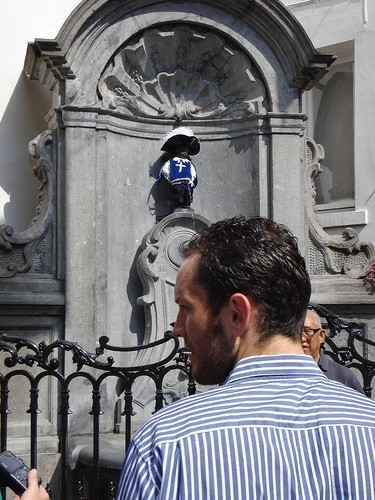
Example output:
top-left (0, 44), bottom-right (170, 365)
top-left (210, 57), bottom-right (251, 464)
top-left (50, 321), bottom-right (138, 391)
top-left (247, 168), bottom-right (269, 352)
top-left (300, 328), bottom-right (321, 336)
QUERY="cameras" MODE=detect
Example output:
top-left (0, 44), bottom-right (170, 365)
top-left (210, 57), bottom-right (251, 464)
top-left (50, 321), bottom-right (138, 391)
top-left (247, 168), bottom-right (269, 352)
top-left (0, 450), bottom-right (42, 496)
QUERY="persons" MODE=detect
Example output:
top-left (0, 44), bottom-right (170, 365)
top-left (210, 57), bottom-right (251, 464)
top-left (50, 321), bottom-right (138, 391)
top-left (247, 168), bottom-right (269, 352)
top-left (154, 124), bottom-right (200, 210)
top-left (302, 309), bottom-right (366, 396)
top-left (116, 215), bottom-right (375, 500)
